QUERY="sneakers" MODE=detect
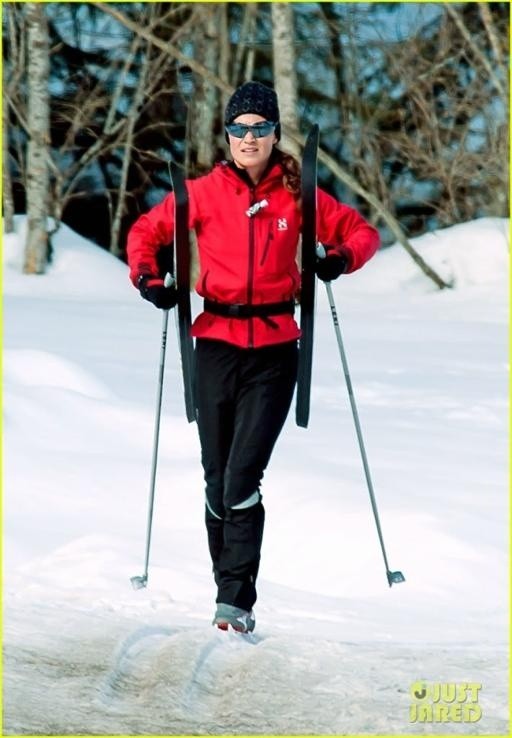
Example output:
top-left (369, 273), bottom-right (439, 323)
top-left (210, 607), bottom-right (255, 631)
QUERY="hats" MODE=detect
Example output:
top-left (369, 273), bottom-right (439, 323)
top-left (225, 83), bottom-right (282, 144)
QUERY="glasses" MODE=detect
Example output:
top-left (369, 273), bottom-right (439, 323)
top-left (224, 120), bottom-right (275, 140)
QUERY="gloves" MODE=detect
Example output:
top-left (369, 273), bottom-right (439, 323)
top-left (317, 255), bottom-right (348, 282)
top-left (142, 285), bottom-right (181, 310)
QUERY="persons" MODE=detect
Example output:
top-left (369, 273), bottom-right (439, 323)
top-left (125, 81), bottom-right (381, 635)
